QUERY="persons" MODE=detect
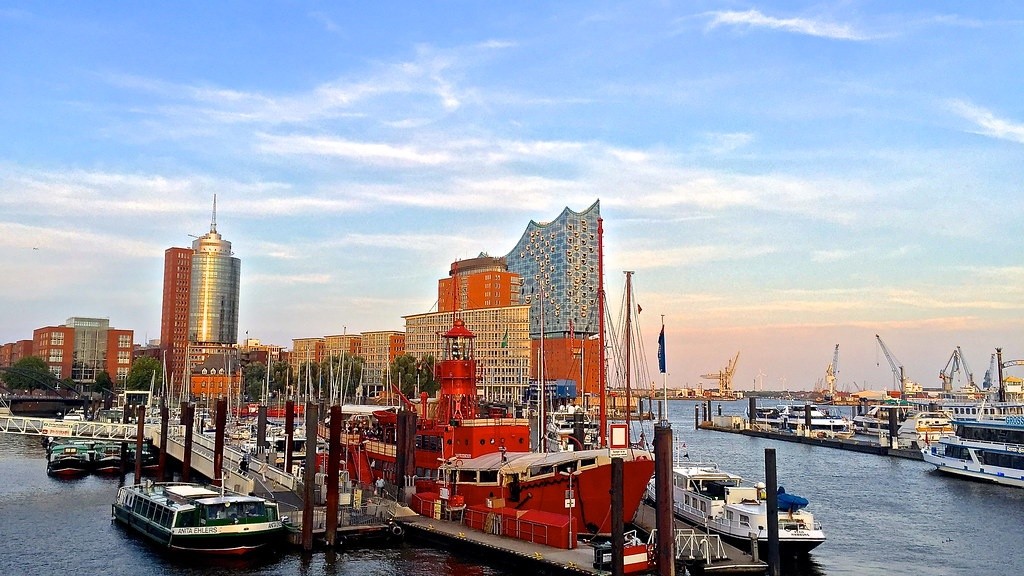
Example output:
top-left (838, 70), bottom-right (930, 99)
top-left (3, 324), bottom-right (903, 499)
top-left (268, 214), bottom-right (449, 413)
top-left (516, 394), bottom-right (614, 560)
top-left (259, 459), bottom-right (269, 482)
top-left (376, 476), bottom-right (385, 497)
top-left (238, 458), bottom-right (246, 476)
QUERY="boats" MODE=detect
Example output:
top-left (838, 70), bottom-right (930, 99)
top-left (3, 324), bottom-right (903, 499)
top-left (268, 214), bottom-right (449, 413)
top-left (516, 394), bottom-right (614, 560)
top-left (851, 405), bottom-right (914, 437)
top-left (113, 482), bottom-right (286, 562)
top-left (744, 407), bottom-right (797, 429)
top-left (123, 437), bottom-right (160, 478)
top-left (777, 404), bottom-right (854, 432)
top-left (41, 437), bottom-right (92, 477)
top-left (86, 440), bottom-right (122, 474)
top-left (643, 433), bottom-right (827, 558)
top-left (895, 409), bottom-right (958, 450)
top-left (921, 418), bottom-right (1024, 488)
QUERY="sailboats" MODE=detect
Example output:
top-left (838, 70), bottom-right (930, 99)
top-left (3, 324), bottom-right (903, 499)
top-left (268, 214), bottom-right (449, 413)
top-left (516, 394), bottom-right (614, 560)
top-left (142, 343), bottom-right (372, 462)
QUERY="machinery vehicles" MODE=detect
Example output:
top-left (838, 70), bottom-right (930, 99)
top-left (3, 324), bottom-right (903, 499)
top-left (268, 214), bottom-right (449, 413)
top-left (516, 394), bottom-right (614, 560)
top-left (875, 334), bottom-right (923, 394)
top-left (956, 344), bottom-right (982, 394)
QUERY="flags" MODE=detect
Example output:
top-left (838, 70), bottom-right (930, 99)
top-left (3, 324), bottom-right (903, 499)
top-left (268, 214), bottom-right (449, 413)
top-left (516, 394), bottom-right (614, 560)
top-left (501, 329), bottom-right (509, 348)
top-left (638, 304), bottom-right (643, 314)
top-left (657, 324), bottom-right (667, 375)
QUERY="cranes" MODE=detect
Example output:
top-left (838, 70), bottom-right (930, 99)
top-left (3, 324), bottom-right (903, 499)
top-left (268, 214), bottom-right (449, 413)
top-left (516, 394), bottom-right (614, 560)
top-left (940, 349), bottom-right (961, 397)
top-left (982, 353), bottom-right (999, 393)
top-left (706, 351), bottom-right (740, 389)
top-left (825, 344), bottom-right (839, 393)
